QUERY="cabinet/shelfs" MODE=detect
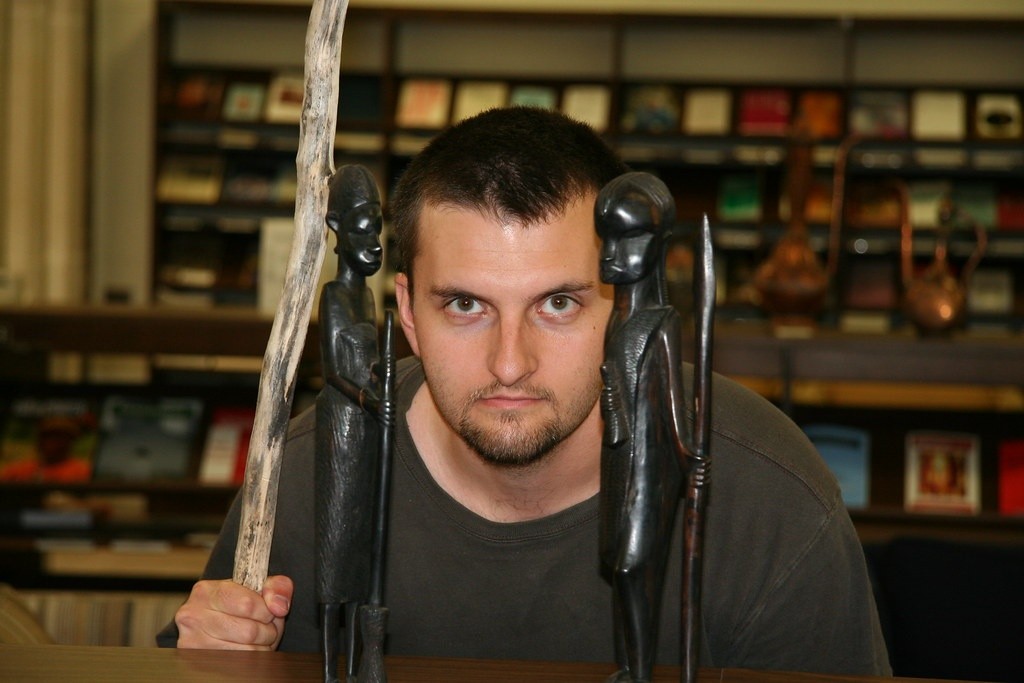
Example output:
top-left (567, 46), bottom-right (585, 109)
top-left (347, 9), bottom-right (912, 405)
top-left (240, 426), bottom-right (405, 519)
top-left (0, 0), bottom-right (1024, 552)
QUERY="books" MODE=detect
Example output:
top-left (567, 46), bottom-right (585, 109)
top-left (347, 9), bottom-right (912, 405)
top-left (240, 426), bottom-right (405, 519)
top-left (0, 77), bottom-right (1023, 576)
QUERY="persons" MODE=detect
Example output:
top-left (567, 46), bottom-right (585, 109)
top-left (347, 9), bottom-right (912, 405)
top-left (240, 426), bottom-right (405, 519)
top-left (154, 107), bottom-right (892, 676)
top-left (593, 172), bottom-right (712, 683)
top-left (312, 164), bottom-right (396, 683)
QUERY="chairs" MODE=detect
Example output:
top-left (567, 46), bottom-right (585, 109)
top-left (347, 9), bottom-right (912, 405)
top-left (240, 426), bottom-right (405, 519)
top-left (874, 534), bottom-right (1024, 683)
